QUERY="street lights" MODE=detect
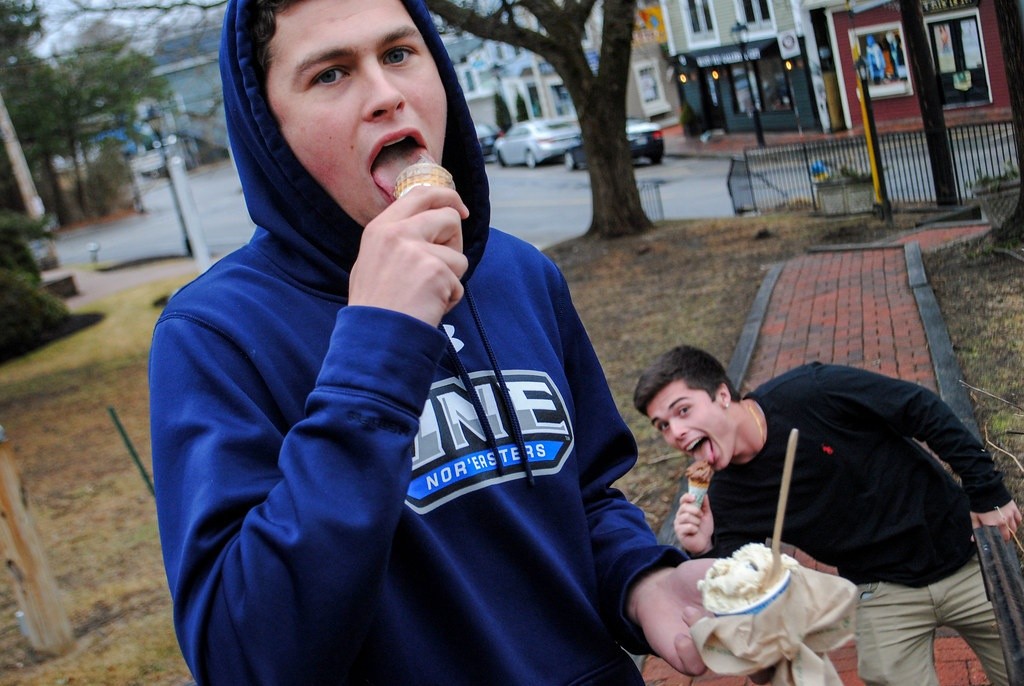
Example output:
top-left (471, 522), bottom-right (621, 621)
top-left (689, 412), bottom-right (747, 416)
top-left (730, 21), bottom-right (766, 149)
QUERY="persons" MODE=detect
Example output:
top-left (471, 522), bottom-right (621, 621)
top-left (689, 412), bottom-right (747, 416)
top-left (631, 341), bottom-right (1021, 686)
top-left (150, 0), bottom-right (733, 686)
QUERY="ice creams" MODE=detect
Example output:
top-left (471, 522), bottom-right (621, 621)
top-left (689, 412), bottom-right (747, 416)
top-left (694, 542), bottom-right (793, 617)
top-left (685, 460), bottom-right (714, 510)
top-left (392, 154), bottom-right (456, 200)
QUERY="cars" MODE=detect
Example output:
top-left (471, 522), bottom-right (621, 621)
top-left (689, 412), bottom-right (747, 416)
top-left (494, 117), bottom-right (583, 168)
top-left (474, 122), bottom-right (499, 160)
top-left (563, 119), bottom-right (664, 171)
